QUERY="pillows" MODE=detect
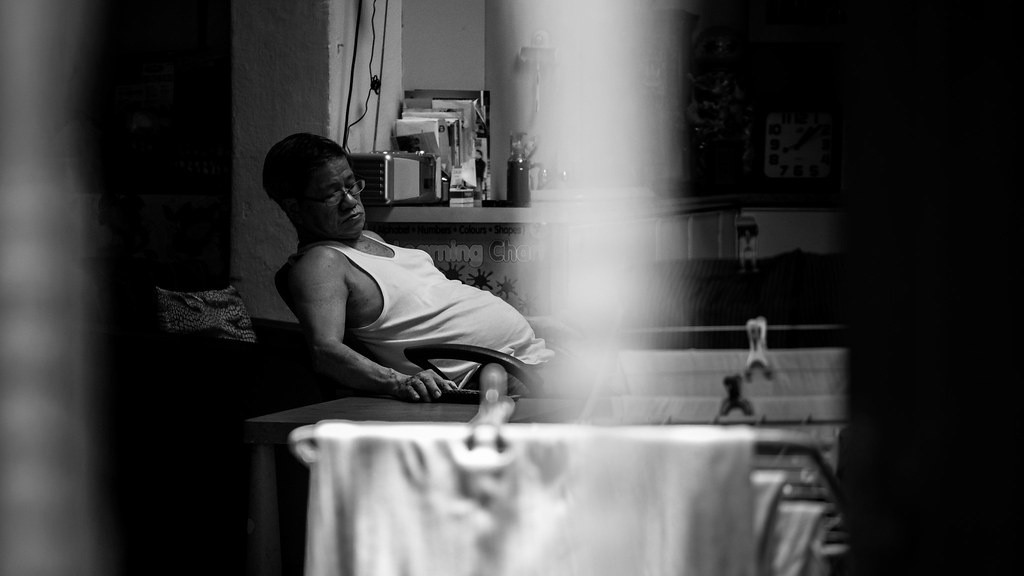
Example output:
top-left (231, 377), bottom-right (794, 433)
top-left (150, 283), bottom-right (257, 342)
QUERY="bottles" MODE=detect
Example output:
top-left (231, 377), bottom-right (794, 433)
top-left (507, 131), bottom-right (530, 206)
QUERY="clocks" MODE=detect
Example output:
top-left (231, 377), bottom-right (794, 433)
top-left (745, 98), bottom-right (843, 197)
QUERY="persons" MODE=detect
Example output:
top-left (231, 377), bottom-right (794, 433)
top-left (475, 150), bottom-right (487, 188)
top-left (262, 132), bottom-right (554, 403)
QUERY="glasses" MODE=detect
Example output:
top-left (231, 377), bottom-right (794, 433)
top-left (303, 180), bottom-right (365, 213)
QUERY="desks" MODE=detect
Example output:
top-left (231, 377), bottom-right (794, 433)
top-left (246, 397), bottom-right (582, 576)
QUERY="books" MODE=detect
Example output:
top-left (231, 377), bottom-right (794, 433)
top-left (396, 97), bottom-right (476, 175)
